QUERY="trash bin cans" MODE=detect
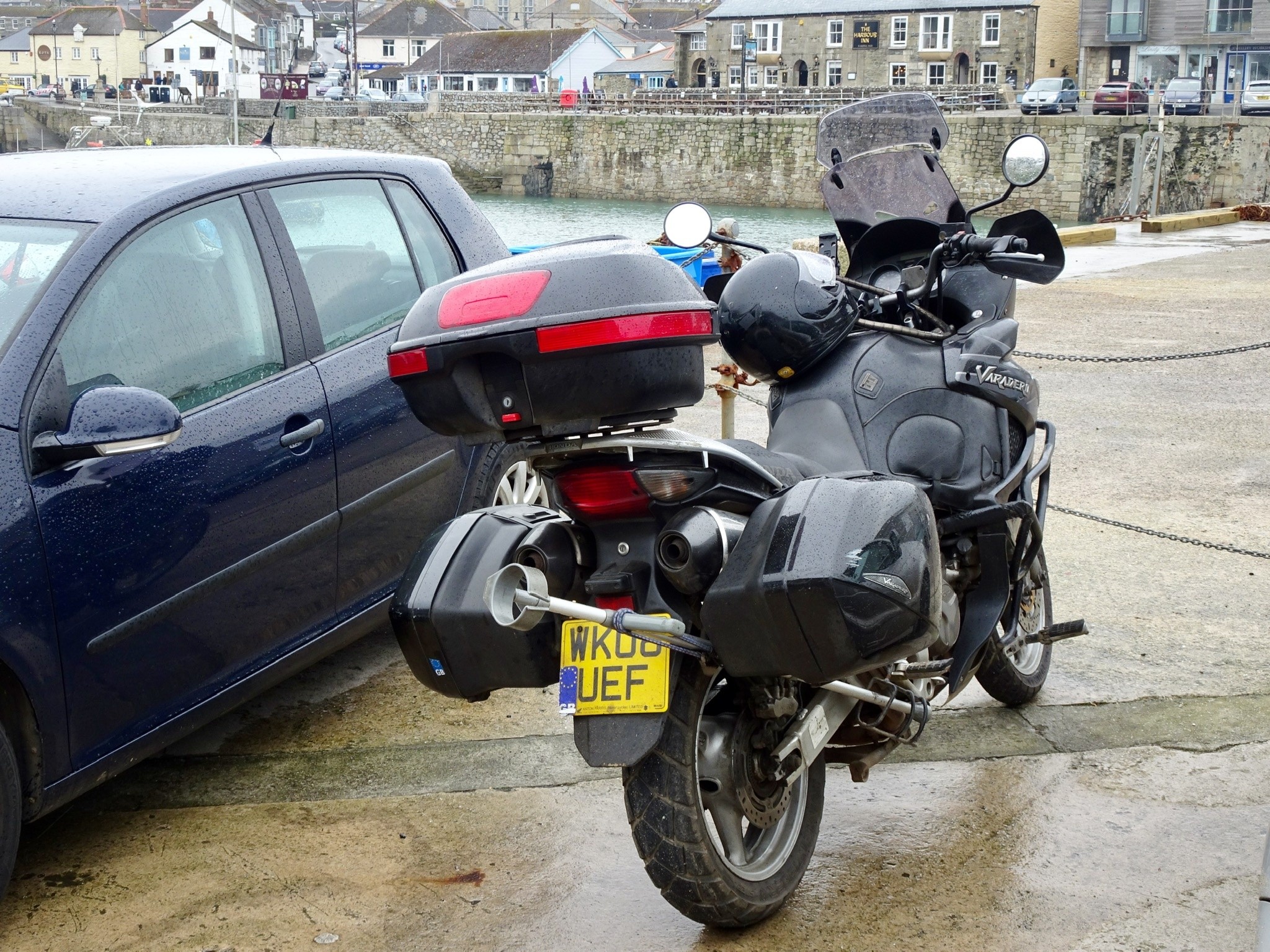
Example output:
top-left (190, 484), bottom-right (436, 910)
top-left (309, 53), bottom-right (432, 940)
top-left (509, 244), bottom-right (714, 294)
top-left (286, 105), bottom-right (295, 119)
top-left (561, 90), bottom-right (578, 113)
top-left (150, 87), bottom-right (160, 102)
top-left (161, 88), bottom-right (170, 103)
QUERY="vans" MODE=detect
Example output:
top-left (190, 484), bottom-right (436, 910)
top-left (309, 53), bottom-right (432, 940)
top-left (1157, 77), bottom-right (1211, 116)
top-left (0, 79), bottom-right (25, 95)
top-left (1021, 77), bottom-right (1080, 115)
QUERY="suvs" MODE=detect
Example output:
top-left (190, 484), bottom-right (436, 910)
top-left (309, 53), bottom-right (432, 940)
top-left (309, 61), bottom-right (327, 78)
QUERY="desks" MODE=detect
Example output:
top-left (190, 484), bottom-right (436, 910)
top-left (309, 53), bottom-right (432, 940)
top-left (936, 93), bottom-right (997, 111)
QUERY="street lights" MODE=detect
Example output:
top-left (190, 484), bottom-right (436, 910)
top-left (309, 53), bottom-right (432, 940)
top-left (51, 23), bottom-right (58, 94)
top-left (95, 56), bottom-right (102, 80)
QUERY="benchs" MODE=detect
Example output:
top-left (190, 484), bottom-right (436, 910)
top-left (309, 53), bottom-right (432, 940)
top-left (982, 99), bottom-right (1001, 102)
top-left (120, 91), bottom-right (145, 103)
top-left (199, 248), bottom-right (406, 337)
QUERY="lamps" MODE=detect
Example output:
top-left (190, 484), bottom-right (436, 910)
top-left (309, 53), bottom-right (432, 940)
top-left (708, 55), bottom-right (714, 66)
top-left (975, 49), bottom-right (980, 62)
top-left (813, 54), bottom-right (819, 66)
top-left (778, 55), bottom-right (783, 66)
top-left (1014, 49), bottom-right (1020, 61)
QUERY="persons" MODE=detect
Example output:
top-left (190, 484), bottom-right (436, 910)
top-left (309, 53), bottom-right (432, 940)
top-left (135, 79), bottom-right (144, 98)
top-left (119, 80), bottom-right (132, 90)
top-left (154, 73), bottom-right (173, 86)
top-left (666, 74), bottom-right (679, 89)
top-left (688, 74), bottom-right (700, 89)
top-left (71, 82), bottom-right (79, 99)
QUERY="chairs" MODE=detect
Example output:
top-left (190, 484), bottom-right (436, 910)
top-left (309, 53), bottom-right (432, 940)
top-left (93, 253), bottom-right (247, 396)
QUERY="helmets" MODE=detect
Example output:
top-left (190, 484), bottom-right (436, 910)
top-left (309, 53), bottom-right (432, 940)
top-left (717, 249), bottom-right (860, 390)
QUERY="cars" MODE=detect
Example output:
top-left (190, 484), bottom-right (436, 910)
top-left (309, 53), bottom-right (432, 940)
top-left (73, 84), bottom-right (117, 98)
top-left (27, 83), bottom-right (66, 100)
top-left (392, 92), bottom-right (424, 103)
top-left (356, 88), bottom-right (392, 102)
top-left (316, 80), bottom-right (334, 96)
top-left (323, 86), bottom-right (350, 100)
top-left (334, 30), bottom-right (352, 55)
top-left (1, 144), bottom-right (560, 902)
top-left (1240, 80), bottom-right (1270, 116)
top-left (1092, 80), bottom-right (1149, 116)
top-left (323, 59), bottom-right (350, 87)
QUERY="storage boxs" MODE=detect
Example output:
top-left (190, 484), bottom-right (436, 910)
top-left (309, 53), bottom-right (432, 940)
top-left (391, 502), bottom-right (587, 703)
top-left (387, 239), bottom-right (721, 437)
top-left (705, 475), bottom-right (943, 688)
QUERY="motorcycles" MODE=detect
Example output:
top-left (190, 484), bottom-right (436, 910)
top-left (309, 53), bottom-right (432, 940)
top-left (387, 88), bottom-right (1091, 931)
